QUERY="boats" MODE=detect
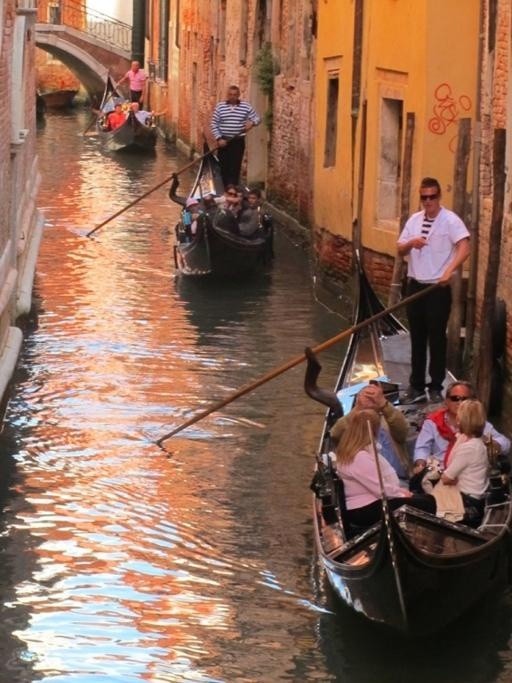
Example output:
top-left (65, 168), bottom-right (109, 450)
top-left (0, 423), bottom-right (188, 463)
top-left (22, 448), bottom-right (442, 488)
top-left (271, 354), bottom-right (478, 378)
top-left (96, 67), bottom-right (158, 152)
top-left (36, 86), bottom-right (80, 108)
top-left (173, 131), bottom-right (272, 276)
top-left (310, 219), bottom-right (512, 632)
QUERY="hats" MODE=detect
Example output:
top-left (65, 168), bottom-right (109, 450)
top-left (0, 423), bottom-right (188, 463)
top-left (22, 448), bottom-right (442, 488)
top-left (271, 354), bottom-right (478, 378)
top-left (186, 198), bottom-right (199, 207)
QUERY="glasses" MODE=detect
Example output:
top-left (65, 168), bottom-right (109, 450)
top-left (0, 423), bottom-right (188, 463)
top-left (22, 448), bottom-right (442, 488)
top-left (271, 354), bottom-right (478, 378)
top-left (228, 192), bottom-right (237, 197)
top-left (451, 395), bottom-right (466, 401)
top-left (421, 195), bottom-right (437, 200)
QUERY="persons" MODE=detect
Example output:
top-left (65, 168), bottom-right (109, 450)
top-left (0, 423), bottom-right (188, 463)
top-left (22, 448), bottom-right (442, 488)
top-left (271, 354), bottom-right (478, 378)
top-left (395, 178), bottom-right (470, 406)
top-left (328, 380), bottom-right (511, 537)
top-left (181, 86), bottom-right (262, 235)
top-left (103, 60), bottom-right (168, 131)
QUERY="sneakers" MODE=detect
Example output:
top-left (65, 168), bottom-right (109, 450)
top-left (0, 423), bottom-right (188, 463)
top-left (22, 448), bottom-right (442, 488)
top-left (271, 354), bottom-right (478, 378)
top-left (400, 387), bottom-right (443, 404)
